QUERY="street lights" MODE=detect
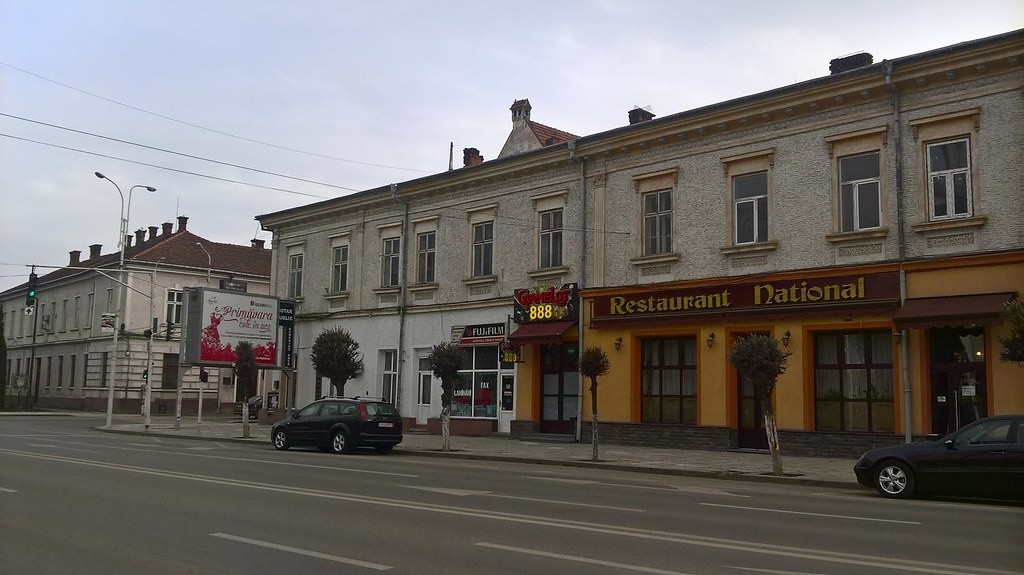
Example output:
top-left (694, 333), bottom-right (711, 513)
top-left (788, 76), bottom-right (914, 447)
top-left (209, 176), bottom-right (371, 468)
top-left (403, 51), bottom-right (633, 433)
top-left (193, 240), bottom-right (212, 423)
top-left (144, 257), bottom-right (165, 427)
top-left (94, 169), bottom-right (157, 428)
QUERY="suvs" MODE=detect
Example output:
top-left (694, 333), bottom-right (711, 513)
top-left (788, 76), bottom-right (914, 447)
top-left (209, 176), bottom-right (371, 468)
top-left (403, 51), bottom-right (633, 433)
top-left (270, 395), bottom-right (404, 456)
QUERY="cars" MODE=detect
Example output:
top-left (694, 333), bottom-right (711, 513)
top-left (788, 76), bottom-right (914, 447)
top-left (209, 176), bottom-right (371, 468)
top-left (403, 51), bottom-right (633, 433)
top-left (233, 396), bottom-right (271, 419)
top-left (852, 414), bottom-right (1023, 503)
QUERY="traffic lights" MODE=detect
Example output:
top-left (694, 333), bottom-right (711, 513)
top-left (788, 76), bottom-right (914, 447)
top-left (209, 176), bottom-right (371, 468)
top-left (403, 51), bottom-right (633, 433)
top-left (143, 370), bottom-right (148, 379)
top-left (25, 273), bottom-right (38, 306)
